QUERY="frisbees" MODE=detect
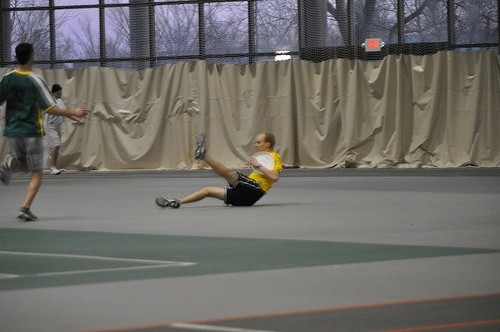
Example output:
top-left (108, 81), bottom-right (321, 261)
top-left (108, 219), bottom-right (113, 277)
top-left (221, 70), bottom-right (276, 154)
top-left (251, 151), bottom-right (275, 175)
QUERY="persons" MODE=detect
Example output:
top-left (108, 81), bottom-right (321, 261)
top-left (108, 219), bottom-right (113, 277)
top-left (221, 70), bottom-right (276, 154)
top-left (0, 43), bottom-right (92, 222)
top-left (42, 80), bottom-right (85, 176)
top-left (154, 131), bottom-right (284, 210)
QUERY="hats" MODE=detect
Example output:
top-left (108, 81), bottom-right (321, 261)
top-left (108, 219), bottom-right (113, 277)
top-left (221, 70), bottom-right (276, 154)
top-left (52, 84), bottom-right (62, 93)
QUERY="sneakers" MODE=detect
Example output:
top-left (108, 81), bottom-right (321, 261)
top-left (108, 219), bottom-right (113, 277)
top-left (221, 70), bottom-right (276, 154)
top-left (0, 164), bottom-right (11, 184)
top-left (17, 208), bottom-right (37, 221)
top-left (49, 166), bottom-right (61, 175)
top-left (156, 197), bottom-right (181, 208)
top-left (195, 135), bottom-right (206, 161)
